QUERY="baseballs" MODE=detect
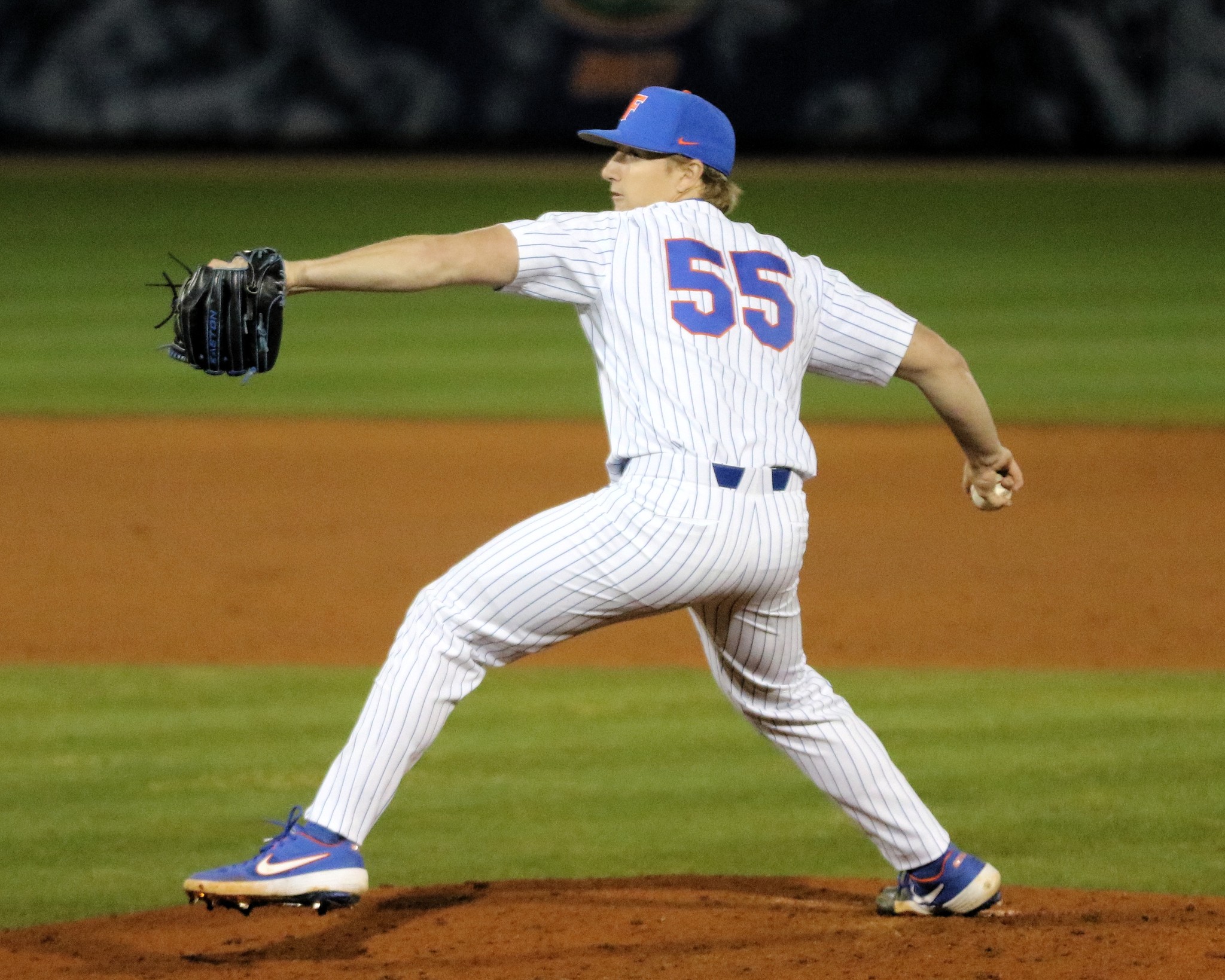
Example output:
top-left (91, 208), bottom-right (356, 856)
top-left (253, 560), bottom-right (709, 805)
top-left (970, 471), bottom-right (1009, 511)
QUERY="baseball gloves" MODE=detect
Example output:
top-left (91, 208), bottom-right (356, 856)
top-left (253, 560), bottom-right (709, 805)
top-left (168, 245), bottom-right (285, 378)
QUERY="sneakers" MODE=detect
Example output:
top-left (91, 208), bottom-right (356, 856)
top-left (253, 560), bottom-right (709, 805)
top-left (183, 806), bottom-right (368, 917)
top-left (876, 846), bottom-right (1002, 918)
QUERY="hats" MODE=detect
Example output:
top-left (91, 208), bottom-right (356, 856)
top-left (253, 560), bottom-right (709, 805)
top-left (578, 86), bottom-right (736, 177)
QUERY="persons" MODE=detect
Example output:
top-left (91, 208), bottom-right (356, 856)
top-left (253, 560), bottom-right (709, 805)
top-left (144, 85), bottom-right (1023, 915)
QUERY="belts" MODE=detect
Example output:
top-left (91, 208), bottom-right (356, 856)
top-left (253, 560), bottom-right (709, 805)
top-left (712, 463), bottom-right (791, 492)
